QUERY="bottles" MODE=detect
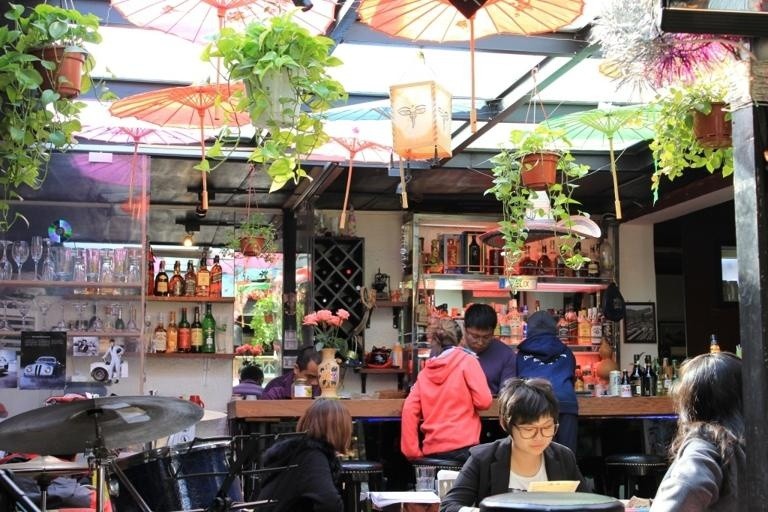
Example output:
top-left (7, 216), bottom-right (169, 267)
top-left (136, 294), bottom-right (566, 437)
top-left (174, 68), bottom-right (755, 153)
top-left (391, 342), bottom-right (404, 368)
top-left (347, 207), bottom-right (357, 235)
top-left (290, 378), bottom-right (311, 398)
top-left (420, 235), bottom-right (600, 277)
top-left (421, 296), bottom-right (605, 346)
top-left (578, 355), bottom-right (678, 397)
top-left (709, 334), bottom-right (720, 352)
top-left (146, 253), bottom-right (222, 352)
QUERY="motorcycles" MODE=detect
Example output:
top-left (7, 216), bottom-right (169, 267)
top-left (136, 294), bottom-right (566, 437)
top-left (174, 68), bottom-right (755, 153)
top-left (76, 341), bottom-right (88, 352)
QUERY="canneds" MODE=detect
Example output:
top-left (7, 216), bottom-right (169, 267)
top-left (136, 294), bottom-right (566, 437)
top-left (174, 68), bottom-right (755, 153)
top-left (609, 371), bottom-right (621, 396)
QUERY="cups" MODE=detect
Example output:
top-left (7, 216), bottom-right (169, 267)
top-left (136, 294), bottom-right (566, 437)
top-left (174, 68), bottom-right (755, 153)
top-left (416, 464), bottom-right (435, 491)
top-left (189, 395), bottom-right (205, 408)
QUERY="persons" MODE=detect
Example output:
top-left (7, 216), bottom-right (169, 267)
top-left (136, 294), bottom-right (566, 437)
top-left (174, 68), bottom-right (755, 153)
top-left (78, 339), bottom-right (88, 352)
top-left (102, 339), bottom-right (124, 384)
top-left (261, 346), bottom-right (323, 399)
top-left (402, 316), bottom-right (493, 463)
top-left (254, 397), bottom-right (353, 512)
top-left (516, 310), bottom-right (581, 454)
top-left (628, 351), bottom-right (745, 512)
top-left (430, 304), bottom-right (517, 399)
top-left (440, 377), bottom-right (588, 512)
top-left (234, 365), bottom-right (265, 396)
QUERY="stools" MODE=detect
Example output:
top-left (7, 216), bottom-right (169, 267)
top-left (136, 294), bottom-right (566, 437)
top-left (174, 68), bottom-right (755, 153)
top-left (600, 449), bottom-right (662, 500)
top-left (334, 459), bottom-right (382, 491)
top-left (409, 456), bottom-right (463, 474)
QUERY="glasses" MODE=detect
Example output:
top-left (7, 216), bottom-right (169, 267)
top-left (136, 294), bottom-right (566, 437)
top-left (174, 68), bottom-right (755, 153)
top-left (511, 418), bottom-right (560, 441)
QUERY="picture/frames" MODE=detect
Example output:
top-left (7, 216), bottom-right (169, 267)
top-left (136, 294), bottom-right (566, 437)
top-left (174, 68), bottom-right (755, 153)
top-left (622, 301), bottom-right (657, 344)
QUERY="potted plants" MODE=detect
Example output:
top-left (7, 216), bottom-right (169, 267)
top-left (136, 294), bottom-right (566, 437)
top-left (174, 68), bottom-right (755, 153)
top-left (607, 70), bottom-right (732, 196)
top-left (484, 127), bottom-right (610, 296)
top-left (1, 2), bottom-right (102, 237)
top-left (184, 16), bottom-right (340, 197)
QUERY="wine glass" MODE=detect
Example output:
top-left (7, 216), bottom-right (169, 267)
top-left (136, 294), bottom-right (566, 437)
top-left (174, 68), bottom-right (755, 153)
top-left (1, 235), bottom-right (140, 331)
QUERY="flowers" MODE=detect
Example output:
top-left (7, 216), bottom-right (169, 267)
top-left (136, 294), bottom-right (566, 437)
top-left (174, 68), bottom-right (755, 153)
top-left (302, 307), bottom-right (351, 350)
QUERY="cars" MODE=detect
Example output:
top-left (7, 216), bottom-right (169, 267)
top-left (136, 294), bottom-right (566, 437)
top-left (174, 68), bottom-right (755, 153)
top-left (25, 356), bottom-right (64, 378)
top-left (88, 358), bottom-right (128, 381)
top-left (0, 355), bottom-right (9, 373)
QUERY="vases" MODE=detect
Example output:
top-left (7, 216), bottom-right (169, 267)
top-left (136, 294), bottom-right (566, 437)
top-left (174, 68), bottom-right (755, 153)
top-left (318, 347), bottom-right (341, 399)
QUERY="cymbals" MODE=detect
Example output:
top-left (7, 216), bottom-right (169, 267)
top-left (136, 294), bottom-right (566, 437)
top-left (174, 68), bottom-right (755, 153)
top-left (0, 455), bottom-right (100, 480)
top-left (0, 396), bottom-right (204, 455)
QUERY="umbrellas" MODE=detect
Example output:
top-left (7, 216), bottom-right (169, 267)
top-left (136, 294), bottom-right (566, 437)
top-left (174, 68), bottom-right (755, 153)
top-left (529, 101), bottom-right (667, 219)
top-left (357, 1), bottom-right (586, 133)
top-left (313, 94), bottom-right (486, 207)
top-left (279, 125), bottom-right (422, 227)
top-left (69, 149), bottom-right (148, 190)
top-left (108, 77), bottom-right (256, 212)
top-left (110, 1), bottom-right (337, 84)
top-left (59, 118), bottom-right (219, 210)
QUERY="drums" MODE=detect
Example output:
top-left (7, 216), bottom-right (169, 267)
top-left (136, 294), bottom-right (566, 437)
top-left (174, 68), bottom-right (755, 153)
top-left (102, 440), bottom-right (244, 512)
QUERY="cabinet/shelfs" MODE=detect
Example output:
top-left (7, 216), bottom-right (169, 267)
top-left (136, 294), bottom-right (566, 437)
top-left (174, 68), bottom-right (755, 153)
top-left (22, 285), bottom-right (236, 362)
top-left (390, 219), bottom-right (618, 361)
